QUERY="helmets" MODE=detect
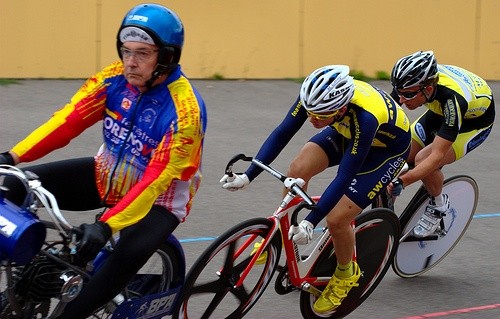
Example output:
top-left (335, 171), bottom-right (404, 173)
top-left (299, 65), bottom-right (355, 113)
top-left (390, 50), bottom-right (438, 89)
top-left (116, 2), bottom-right (185, 66)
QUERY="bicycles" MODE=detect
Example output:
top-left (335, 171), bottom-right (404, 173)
top-left (0, 164), bottom-right (187, 319)
top-left (172, 153), bottom-right (401, 319)
top-left (359, 174), bottom-right (480, 278)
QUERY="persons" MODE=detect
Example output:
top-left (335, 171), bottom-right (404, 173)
top-left (378, 51), bottom-right (496, 237)
top-left (220, 65), bottom-right (412, 314)
top-left (0, 4), bottom-right (208, 319)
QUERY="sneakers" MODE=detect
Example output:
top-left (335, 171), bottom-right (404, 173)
top-left (413, 194), bottom-right (450, 237)
top-left (250, 224), bottom-right (284, 265)
top-left (313, 261), bottom-right (361, 316)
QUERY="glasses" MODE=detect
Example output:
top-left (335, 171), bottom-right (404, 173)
top-left (120, 46), bottom-right (158, 61)
top-left (305, 110), bottom-right (339, 121)
top-left (394, 84), bottom-right (433, 100)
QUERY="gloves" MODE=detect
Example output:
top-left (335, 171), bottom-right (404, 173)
top-left (288, 219), bottom-right (314, 245)
top-left (75, 222), bottom-right (112, 262)
top-left (0, 151), bottom-right (15, 169)
top-left (219, 172), bottom-right (250, 192)
